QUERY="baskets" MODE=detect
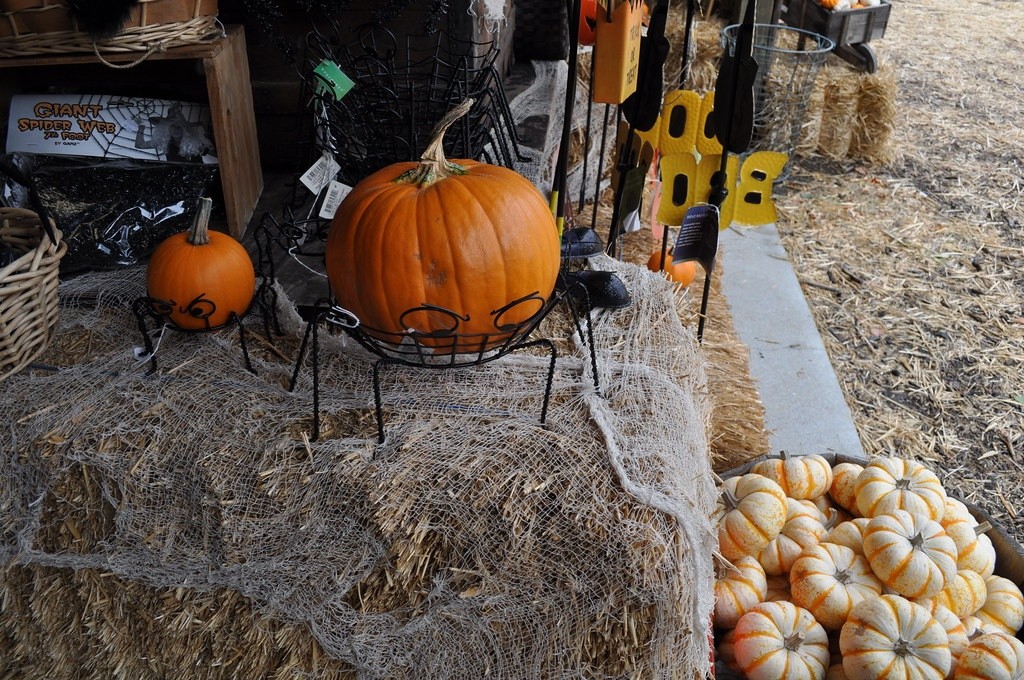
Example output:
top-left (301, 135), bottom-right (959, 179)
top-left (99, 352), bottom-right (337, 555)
top-left (1, 154), bottom-right (71, 382)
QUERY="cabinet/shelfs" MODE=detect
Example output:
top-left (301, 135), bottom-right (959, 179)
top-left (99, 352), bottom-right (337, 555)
top-left (1, 23), bottom-right (265, 243)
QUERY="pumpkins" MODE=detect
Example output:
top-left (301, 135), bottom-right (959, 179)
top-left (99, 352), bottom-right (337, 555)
top-left (147, 197), bottom-right (255, 333)
top-left (647, 246), bottom-right (697, 289)
top-left (325, 96), bottom-right (560, 355)
top-left (710, 449), bottom-right (1024, 680)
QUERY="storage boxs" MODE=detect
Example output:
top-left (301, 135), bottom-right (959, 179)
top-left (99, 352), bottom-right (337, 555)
top-left (592, 0), bottom-right (644, 104)
top-left (714, 451), bottom-right (1023, 593)
top-left (782, 0), bottom-right (892, 47)
